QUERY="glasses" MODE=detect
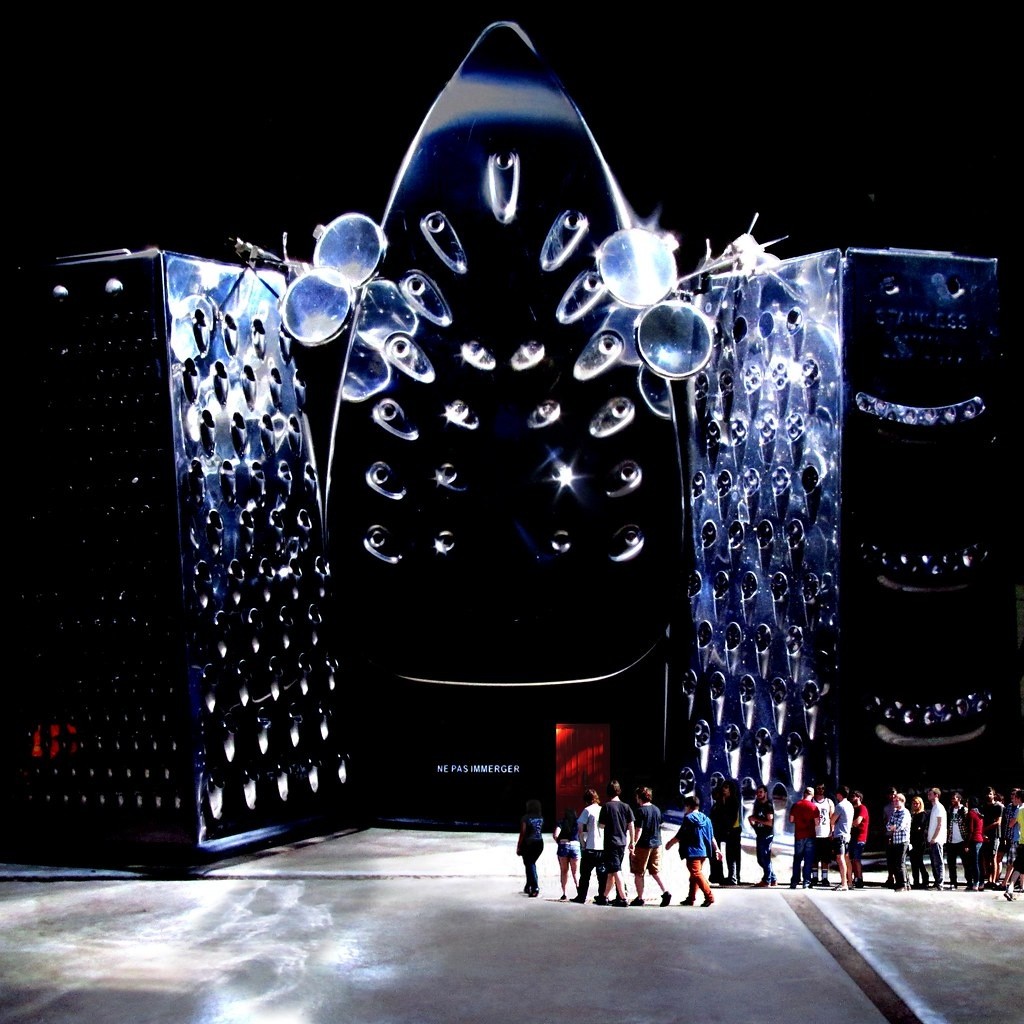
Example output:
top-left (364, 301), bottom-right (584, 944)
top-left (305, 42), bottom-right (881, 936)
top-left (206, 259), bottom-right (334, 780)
top-left (816, 787), bottom-right (825, 790)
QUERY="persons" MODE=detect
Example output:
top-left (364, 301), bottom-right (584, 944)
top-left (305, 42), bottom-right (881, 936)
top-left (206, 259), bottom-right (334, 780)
top-left (852, 791), bottom-right (869, 888)
top-left (703, 781), bottom-right (755, 888)
top-left (628, 785), bottom-right (671, 907)
top-left (747, 785), bottom-right (777, 887)
top-left (831, 786), bottom-right (854, 891)
top-left (807, 782), bottom-right (835, 889)
top-left (570, 789), bottom-right (607, 906)
top-left (881, 786), bottom-right (1024, 901)
top-left (553, 809), bottom-right (579, 902)
top-left (666, 796), bottom-right (723, 907)
top-left (886, 793), bottom-right (911, 892)
top-left (965, 796), bottom-right (985, 891)
top-left (927, 788), bottom-right (947, 891)
top-left (910, 796), bottom-right (929, 890)
top-left (517, 800), bottom-right (543, 897)
top-left (789, 786), bottom-right (820, 889)
top-left (594, 779), bottom-right (636, 907)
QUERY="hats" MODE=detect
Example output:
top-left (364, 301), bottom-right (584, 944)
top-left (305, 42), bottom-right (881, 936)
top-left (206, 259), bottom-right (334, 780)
top-left (932, 788), bottom-right (942, 799)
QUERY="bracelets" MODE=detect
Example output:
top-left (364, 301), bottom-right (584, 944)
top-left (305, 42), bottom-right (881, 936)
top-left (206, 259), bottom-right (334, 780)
top-left (715, 850), bottom-right (720, 855)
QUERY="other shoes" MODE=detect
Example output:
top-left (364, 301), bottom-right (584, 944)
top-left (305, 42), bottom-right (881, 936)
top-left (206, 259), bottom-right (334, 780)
top-left (701, 899), bottom-right (713, 907)
top-left (948, 884), bottom-right (957, 889)
top-left (853, 881), bottom-right (863, 888)
top-left (660, 891), bottom-right (671, 907)
top-left (524, 887), bottom-right (530, 894)
top-left (881, 880), bottom-right (897, 889)
top-left (720, 879), bottom-right (731, 885)
top-left (570, 895), bottom-right (586, 904)
top-left (531, 888), bottom-right (539, 898)
top-left (1004, 891), bottom-right (1015, 901)
top-left (803, 884), bottom-right (813, 889)
top-left (965, 881), bottom-right (1023, 893)
top-left (680, 899), bottom-right (694, 906)
top-left (833, 886), bottom-right (853, 891)
top-left (755, 881), bottom-right (777, 886)
top-left (894, 882), bottom-right (944, 892)
top-left (790, 884), bottom-right (796, 888)
top-left (561, 895), bottom-right (566, 899)
top-left (592, 896), bottom-right (644, 907)
top-left (812, 878), bottom-right (831, 887)
